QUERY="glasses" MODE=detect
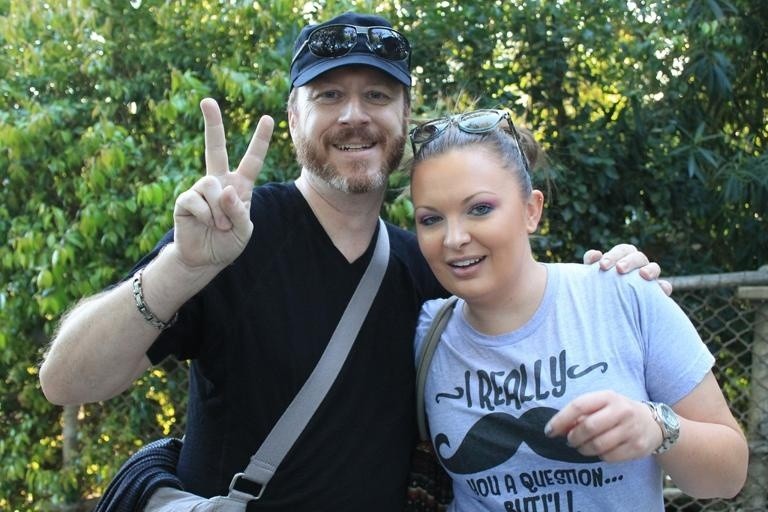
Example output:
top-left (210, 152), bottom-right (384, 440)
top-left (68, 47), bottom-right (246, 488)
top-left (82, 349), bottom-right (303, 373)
top-left (292, 26), bottom-right (409, 66)
top-left (410, 108), bottom-right (531, 171)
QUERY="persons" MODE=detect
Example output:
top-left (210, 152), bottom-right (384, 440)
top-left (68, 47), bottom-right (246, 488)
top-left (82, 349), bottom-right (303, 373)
top-left (39, 12), bottom-right (674, 511)
top-left (411, 106), bottom-right (750, 512)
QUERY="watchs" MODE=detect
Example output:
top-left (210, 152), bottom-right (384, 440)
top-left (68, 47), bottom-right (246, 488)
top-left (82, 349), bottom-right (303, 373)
top-left (642, 397), bottom-right (680, 455)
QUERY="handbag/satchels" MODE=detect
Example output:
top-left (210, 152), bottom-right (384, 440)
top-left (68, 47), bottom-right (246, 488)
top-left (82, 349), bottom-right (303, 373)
top-left (402, 439), bottom-right (455, 511)
top-left (96, 438), bottom-right (249, 512)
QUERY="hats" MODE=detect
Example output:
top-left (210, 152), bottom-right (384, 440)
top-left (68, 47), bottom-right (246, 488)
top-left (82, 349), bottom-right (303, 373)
top-left (291, 12), bottom-right (411, 89)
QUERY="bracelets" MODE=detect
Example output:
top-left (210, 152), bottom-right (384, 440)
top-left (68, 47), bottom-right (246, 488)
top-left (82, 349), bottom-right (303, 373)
top-left (131, 268), bottom-right (178, 330)
